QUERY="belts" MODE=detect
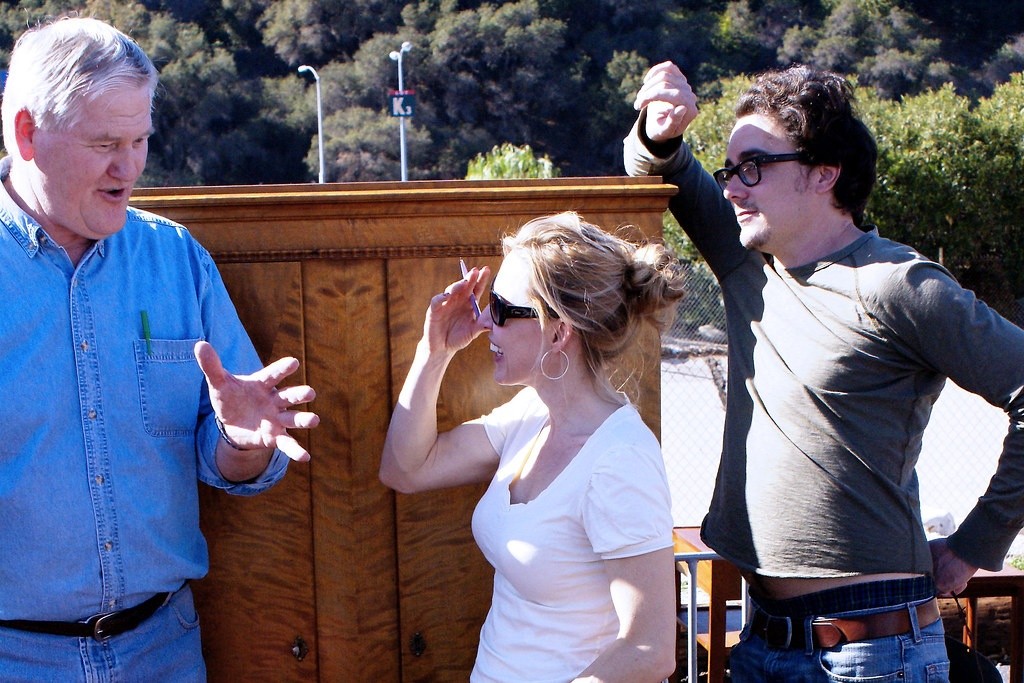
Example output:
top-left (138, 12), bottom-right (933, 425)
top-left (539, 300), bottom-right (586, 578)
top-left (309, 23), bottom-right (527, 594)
top-left (748, 597), bottom-right (940, 651)
top-left (0, 591), bottom-right (169, 643)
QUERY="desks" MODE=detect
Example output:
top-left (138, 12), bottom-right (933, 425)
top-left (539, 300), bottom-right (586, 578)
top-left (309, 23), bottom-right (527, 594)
top-left (673, 525), bottom-right (1024, 683)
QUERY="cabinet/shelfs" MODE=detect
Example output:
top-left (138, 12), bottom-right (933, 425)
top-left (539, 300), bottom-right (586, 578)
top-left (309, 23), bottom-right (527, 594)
top-left (130, 174), bottom-right (685, 683)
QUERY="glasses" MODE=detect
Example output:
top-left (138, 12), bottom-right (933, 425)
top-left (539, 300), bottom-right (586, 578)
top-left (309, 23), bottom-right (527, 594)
top-left (489, 276), bottom-right (562, 327)
top-left (713, 152), bottom-right (806, 192)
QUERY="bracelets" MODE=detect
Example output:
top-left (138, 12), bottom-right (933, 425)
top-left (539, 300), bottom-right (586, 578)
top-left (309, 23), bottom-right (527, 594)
top-left (214, 413), bottom-right (248, 453)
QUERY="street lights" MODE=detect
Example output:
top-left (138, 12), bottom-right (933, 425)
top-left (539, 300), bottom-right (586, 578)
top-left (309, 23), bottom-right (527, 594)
top-left (298, 65), bottom-right (324, 184)
top-left (389, 41), bottom-right (413, 181)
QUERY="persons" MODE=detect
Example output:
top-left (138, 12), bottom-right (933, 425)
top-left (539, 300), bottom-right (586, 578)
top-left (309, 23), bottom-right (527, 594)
top-left (377, 210), bottom-right (686, 683)
top-left (622, 60), bottom-right (1024, 683)
top-left (0, 17), bottom-right (321, 683)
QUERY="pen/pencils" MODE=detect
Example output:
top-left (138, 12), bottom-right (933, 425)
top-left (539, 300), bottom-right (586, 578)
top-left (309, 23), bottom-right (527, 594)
top-left (140, 308), bottom-right (155, 352)
top-left (459, 256), bottom-right (481, 318)
